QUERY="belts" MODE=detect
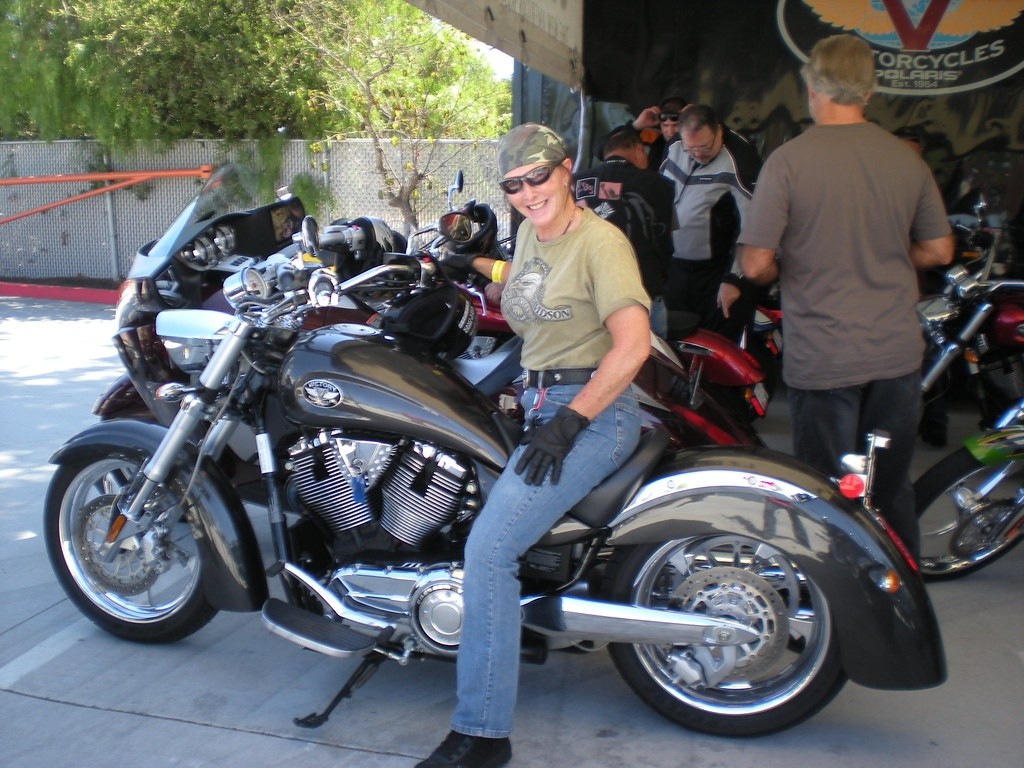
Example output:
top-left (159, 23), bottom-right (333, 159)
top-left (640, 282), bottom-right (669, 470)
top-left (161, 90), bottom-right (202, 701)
top-left (530, 368), bottom-right (598, 389)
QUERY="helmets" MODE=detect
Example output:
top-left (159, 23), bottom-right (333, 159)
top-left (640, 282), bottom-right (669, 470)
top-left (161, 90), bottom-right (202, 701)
top-left (384, 280), bottom-right (477, 362)
top-left (447, 200), bottom-right (500, 254)
top-left (336, 216), bottom-right (408, 278)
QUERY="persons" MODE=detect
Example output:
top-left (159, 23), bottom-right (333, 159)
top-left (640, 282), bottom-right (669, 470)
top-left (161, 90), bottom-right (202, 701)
top-left (591, 100), bottom-right (693, 171)
top-left (733, 32), bottom-right (957, 585)
top-left (890, 123), bottom-right (933, 167)
top-left (569, 121), bottom-right (680, 338)
top-left (410, 117), bottom-right (655, 767)
top-left (652, 103), bottom-right (765, 348)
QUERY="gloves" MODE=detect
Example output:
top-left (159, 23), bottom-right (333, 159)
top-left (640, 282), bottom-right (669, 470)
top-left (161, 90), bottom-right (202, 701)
top-left (443, 253), bottom-right (486, 281)
top-left (514, 405), bottom-right (590, 486)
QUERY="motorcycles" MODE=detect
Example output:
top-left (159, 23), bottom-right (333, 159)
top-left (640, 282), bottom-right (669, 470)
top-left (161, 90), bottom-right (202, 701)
top-left (42, 158), bottom-right (1024, 738)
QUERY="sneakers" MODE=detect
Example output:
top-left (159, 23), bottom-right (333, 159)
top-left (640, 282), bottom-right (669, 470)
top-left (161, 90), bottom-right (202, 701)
top-left (563, 641), bottom-right (593, 653)
top-left (414, 730), bottom-right (512, 768)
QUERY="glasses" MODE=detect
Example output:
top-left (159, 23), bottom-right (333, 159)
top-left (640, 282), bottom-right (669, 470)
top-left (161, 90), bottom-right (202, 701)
top-left (658, 113), bottom-right (680, 121)
top-left (680, 133), bottom-right (717, 152)
top-left (499, 155), bottom-right (570, 194)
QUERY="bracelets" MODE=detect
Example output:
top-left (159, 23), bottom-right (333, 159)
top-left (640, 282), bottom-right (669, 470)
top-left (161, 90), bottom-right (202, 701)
top-left (491, 258), bottom-right (506, 285)
